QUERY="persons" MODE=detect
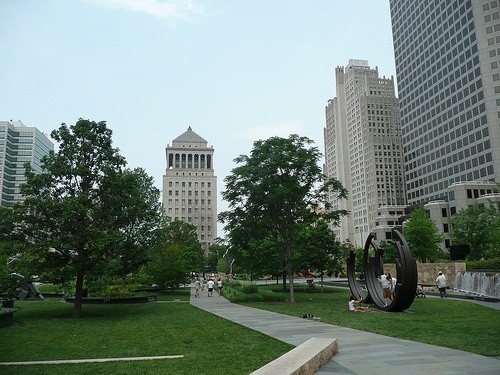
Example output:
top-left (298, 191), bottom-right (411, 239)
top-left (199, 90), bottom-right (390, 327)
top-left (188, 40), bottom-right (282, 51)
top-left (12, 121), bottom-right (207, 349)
top-left (207, 279), bottom-right (214, 297)
top-left (436, 270), bottom-right (448, 298)
top-left (195, 279), bottom-right (200, 298)
top-left (202, 278), bottom-right (207, 293)
top-left (217, 279), bottom-right (222, 294)
top-left (308, 278), bottom-right (315, 289)
top-left (348, 296), bottom-right (371, 313)
top-left (377, 271), bottom-right (397, 307)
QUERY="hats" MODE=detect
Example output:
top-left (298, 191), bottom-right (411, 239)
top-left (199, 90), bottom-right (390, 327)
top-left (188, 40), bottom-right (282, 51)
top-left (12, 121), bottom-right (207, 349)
top-left (385, 273), bottom-right (390, 278)
top-left (438, 271), bottom-right (442, 275)
